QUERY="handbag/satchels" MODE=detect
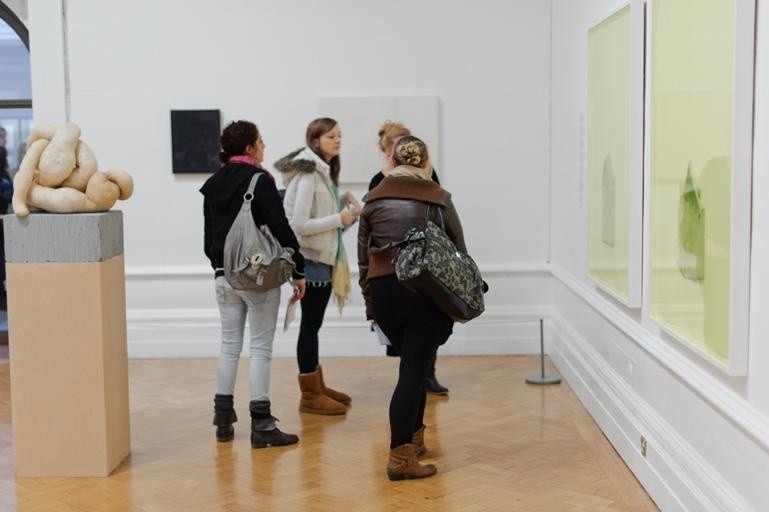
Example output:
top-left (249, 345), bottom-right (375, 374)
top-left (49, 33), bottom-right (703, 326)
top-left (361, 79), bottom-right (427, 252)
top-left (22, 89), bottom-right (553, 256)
top-left (393, 221), bottom-right (489, 325)
top-left (221, 201), bottom-right (295, 293)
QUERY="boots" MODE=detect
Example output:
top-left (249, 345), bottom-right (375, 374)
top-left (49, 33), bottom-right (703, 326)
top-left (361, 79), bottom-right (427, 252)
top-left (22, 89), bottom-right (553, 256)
top-left (297, 365), bottom-right (351, 415)
top-left (411, 425), bottom-right (427, 457)
top-left (386, 443), bottom-right (437, 482)
top-left (250, 401), bottom-right (299, 449)
top-left (214, 395), bottom-right (238, 442)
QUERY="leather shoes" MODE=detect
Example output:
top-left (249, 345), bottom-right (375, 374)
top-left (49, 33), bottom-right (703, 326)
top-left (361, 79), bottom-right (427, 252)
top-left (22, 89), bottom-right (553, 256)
top-left (427, 372), bottom-right (450, 396)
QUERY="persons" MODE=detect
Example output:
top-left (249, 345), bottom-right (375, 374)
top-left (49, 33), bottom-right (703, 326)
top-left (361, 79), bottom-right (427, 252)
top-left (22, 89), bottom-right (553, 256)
top-left (367, 120), bottom-right (450, 396)
top-left (0, 125), bottom-right (14, 312)
top-left (282, 116), bottom-right (362, 417)
top-left (356, 133), bottom-right (490, 484)
top-left (198, 118), bottom-right (307, 449)
